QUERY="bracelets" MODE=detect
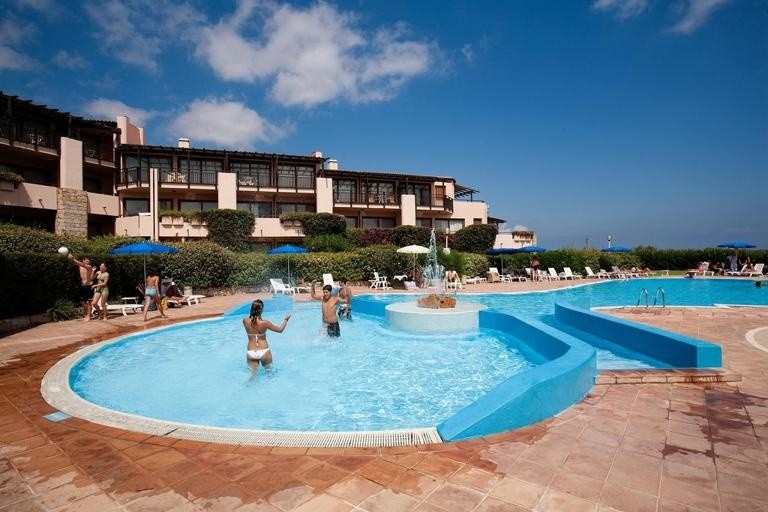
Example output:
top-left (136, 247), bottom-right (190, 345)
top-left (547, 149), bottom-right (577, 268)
top-left (284, 318), bottom-right (288, 321)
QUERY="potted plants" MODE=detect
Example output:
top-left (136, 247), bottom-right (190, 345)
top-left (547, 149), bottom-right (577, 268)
top-left (158, 210), bottom-right (212, 226)
top-left (279, 212), bottom-right (312, 227)
top-left (0, 171), bottom-right (25, 191)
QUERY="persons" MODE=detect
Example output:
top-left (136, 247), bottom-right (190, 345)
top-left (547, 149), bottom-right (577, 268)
top-left (89, 262), bottom-right (109, 322)
top-left (634, 266), bottom-right (653, 273)
top-left (754, 281), bottom-right (762, 287)
top-left (243, 299), bottom-right (291, 380)
top-left (297, 274), bottom-right (311, 288)
top-left (162, 272), bottom-right (189, 301)
top-left (403, 266), bottom-right (414, 281)
top-left (531, 256), bottom-right (539, 271)
top-left (417, 265), bottom-right (427, 278)
top-left (310, 278), bottom-right (348, 338)
top-left (697, 251), bottom-right (755, 276)
top-left (413, 265), bottom-right (426, 289)
top-left (447, 268), bottom-right (460, 283)
top-left (529, 255), bottom-right (540, 282)
top-left (335, 276), bottom-right (353, 322)
top-left (68, 252), bottom-right (93, 322)
top-left (142, 264), bottom-right (168, 320)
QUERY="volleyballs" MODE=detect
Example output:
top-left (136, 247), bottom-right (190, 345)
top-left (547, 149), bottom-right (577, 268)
top-left (57, 246), bottom-right (69, 255)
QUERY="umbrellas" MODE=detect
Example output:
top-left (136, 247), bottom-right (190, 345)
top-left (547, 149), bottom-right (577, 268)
top-left (484, 248), bottom-right (517, 275)
top-left (109, 240), bottom-right (179, 293)
top-left (517, 246), bottom-right (547, 261)
top-left (716, 241), bottom-right (757, 271)
top-left (600, 246), bottom-right (632, 272)
top-left (396, 244), bottom-right (432, 278)
top-left (266, 244), bottom-right (309, 284)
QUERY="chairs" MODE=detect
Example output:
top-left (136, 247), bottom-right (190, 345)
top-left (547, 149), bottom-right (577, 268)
top-left (91, 276), bottom-right (206, 317)
top-left (584, 264), bottom-right (669, 279)
top-left (269, 279), bottom-right (295, 296)
top-left (368, 266), bottom-right (582, 291)
top-left (323, 273), bottom-right (340, 289)
top-left (686, 262), bottom-right (764, 278)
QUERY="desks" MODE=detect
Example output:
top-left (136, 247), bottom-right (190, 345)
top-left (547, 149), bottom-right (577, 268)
top-left (292, 287), bottom-right (308, 294)
